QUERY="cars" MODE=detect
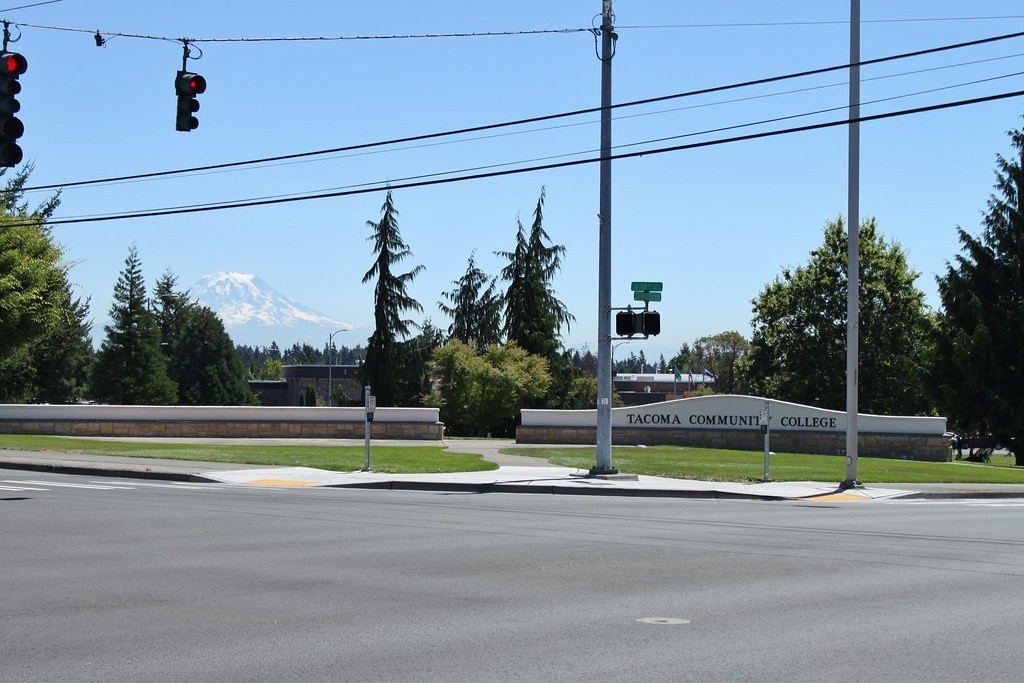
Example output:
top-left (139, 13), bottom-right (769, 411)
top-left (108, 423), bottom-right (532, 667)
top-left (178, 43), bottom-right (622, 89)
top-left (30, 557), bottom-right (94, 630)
top-left (945, 431), bottom-right (1004, 450)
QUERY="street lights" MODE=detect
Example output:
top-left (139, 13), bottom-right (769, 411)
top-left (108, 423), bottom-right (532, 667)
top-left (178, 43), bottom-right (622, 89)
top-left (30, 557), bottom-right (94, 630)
top-left (328, 329), bottom-right (349, 406)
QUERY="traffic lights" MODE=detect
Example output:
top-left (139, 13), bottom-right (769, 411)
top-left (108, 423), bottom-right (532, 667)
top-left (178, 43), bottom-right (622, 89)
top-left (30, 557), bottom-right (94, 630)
top-left (175, 70), bottom-right (206, 131)
top-left (616, 313), bottom-right (638, 336)
top-left (0, 49), bottom-right (28, 166)
top-left (638, 311), bottom-right (661, 336)
